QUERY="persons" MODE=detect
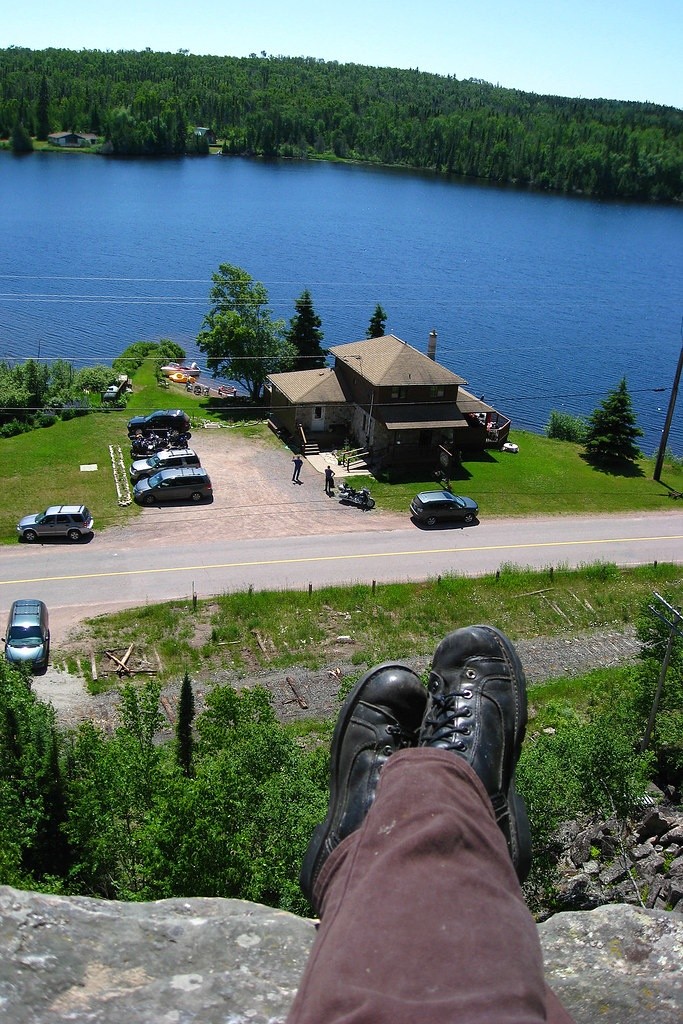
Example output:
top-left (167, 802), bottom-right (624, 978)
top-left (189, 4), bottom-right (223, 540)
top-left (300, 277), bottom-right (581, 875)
top-left (287, 624), bottom-right (576, 1024)
top-left (323, 466), bottom-right (335, 492)
top-left (292, 455), bottom-right (303, 480)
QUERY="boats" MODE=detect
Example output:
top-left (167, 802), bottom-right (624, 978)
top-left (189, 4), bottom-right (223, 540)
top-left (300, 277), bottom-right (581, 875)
top-left (160, 362), bottom-right (201, 378)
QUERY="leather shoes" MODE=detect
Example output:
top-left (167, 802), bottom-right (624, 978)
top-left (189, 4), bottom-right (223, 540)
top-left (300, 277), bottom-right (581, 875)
top-left (299, 661), bottom-right (427, 903)
top-left (417, 625), bottom-right (532, 886)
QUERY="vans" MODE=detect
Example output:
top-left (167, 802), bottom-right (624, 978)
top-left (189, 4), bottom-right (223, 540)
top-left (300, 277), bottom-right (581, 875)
top-left (1, 598), bottom-right (50, 675)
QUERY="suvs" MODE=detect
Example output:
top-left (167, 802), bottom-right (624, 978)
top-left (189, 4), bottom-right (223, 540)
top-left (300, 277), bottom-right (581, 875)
top-left (129, 448), bottom-right (201, 484)
top-left (17, 505), bottom-right (94, 543)
top-left (133, 467), bottom-right (214, 506)
top-left (409, 489), bottom-right (479, 528)
top-left (126, 408), bottom-right (192, 434)
